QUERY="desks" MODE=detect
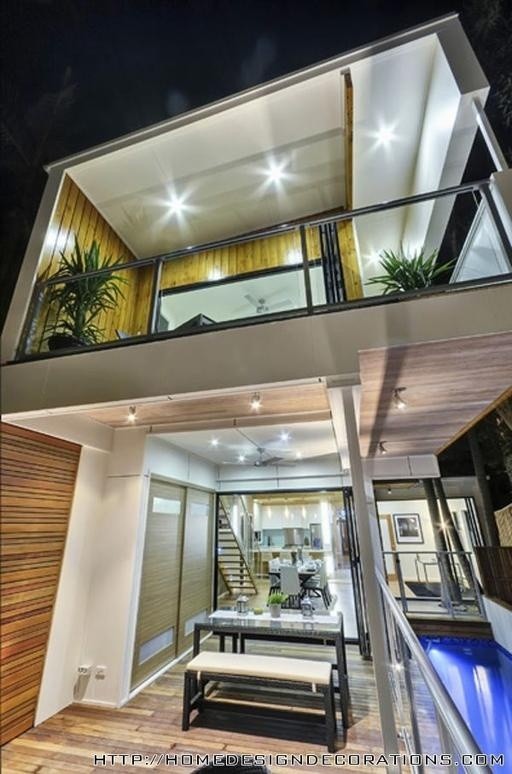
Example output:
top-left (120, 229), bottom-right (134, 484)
top-left (194, 611), bottom-right (350, 728)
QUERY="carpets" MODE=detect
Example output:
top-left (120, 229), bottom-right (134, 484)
top-left (406, 580), bottom-right (442, 597)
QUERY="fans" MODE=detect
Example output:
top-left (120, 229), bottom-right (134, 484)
top-left (222, 447), bottom-right (297, 469)
top-left (245, 294), bottom-right (292, 313)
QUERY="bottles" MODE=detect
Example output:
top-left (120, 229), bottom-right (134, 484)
top-left (300, 596), bottom-right (312, 617)
top-left (236, 595), bottom-right (249, 614)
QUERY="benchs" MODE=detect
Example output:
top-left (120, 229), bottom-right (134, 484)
top-left (183, 651), bottom-right (337, 754)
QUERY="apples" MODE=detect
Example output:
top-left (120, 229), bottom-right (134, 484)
top-left (253, 607), bottom-right (263, 615)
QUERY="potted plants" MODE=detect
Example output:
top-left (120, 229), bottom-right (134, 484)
top-left (32, 231), bottom-right (128, 353)
top-left (266, 591), bottom-right (288, 618)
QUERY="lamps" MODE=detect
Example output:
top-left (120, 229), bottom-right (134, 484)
top-left (127, 405), bottom-right (137, 422)
top-left (376, 441), bottom-right (386, 455)
top-left (250, 391), bottom-right (261, 405)
top-left (392, 387), bottom-right (407, 409)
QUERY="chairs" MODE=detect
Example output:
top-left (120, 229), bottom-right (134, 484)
top-left (268, 557), bottom-right (332, 609)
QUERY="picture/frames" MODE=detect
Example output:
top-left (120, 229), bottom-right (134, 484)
top-left (394, 513), bottom-right (424, 544)
